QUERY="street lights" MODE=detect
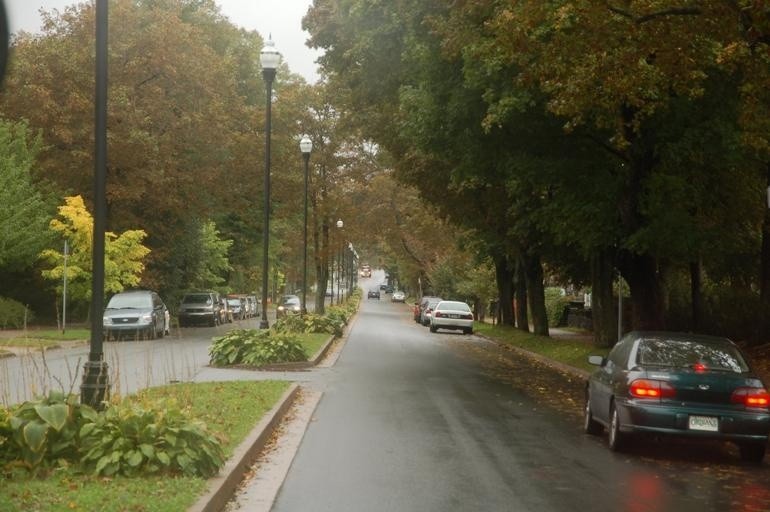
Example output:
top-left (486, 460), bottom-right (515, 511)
top-left (299, 133), bottom-right (313, 317)
top-left (336, 217), bottom-right (361, 306)
top-left (254, 31), bottom-right (285, 328)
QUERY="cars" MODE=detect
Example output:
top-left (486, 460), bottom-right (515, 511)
top-left (361, 263), bottom-right (371, 277)
top-left (380, 284), bottom-right (393, 293)
top-left (325, 286), bottom-right (335, 297)
top-left (583, 329), bottom-right (769, 464)
top-left (412, 296), bottom-right (474, 334)
top-left (391, 291), bottom-right (405, 302)
top-left (368, 288), bottom-right (380, 300)
top-left (104, 289), bottom-right (303, 341)
